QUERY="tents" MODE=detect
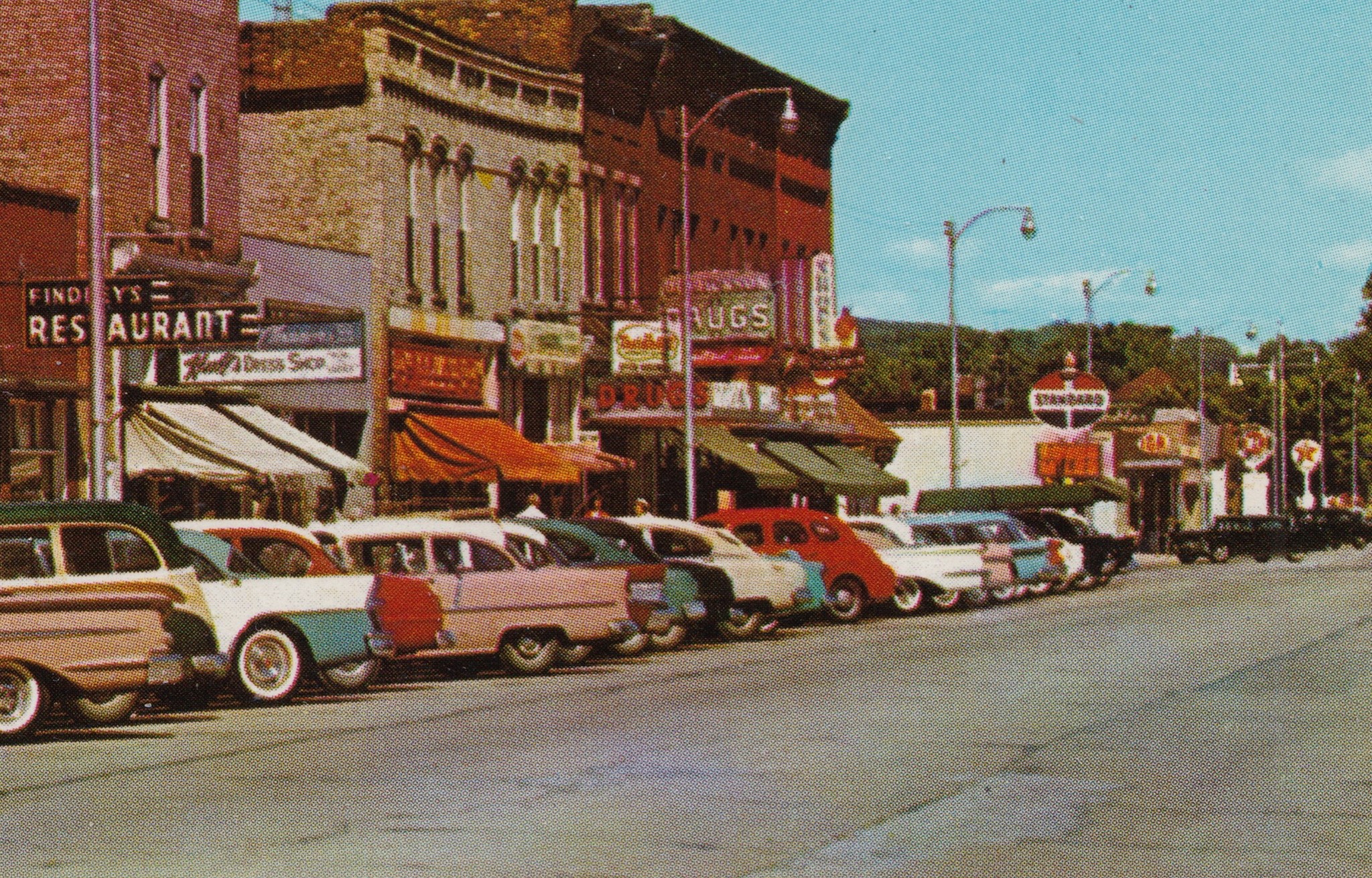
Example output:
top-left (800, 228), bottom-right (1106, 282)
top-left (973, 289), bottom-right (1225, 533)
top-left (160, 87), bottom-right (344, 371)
top-left (390, 402), bottom-right (583, 486)
top-left (916, 478), bottom-right (1131, 513)
top-left (677, 421), bottom-right (799, 491)
top-left (762, 442), bottom-right (908, 496)
top-left (117, 401), bottom-right (374, 493)
top-left (546, 441), bottom-right (639, 475)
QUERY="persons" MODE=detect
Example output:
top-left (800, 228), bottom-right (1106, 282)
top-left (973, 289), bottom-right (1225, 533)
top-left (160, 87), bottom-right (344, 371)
top-left (634, 497), bottom-right (658, 555)
top-left (584, 497), bottom-right (612, 517)
top-left (515, 493), bottom-right (549, 519)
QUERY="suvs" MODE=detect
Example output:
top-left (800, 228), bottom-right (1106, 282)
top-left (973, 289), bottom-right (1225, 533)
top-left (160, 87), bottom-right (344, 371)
top-left (0, 497), bottom-right (228, 746)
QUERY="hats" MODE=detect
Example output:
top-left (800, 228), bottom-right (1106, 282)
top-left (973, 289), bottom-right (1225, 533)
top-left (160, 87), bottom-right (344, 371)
top-left (635, 498), bottom-right (648, 506)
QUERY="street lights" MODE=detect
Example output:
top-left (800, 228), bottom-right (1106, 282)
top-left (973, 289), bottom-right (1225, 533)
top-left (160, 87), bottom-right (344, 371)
top-left (1279, 332), bottom-right (1319, 515)
top-left (943, 206), bottom-right (1037, 488)
top-left (1083, 267), bottom-right (1161, 374)
top-left (1318, 368), bottom-right (1362, 505)
top-left (679, 86), bottom-right (800, 520)
top-left (1194, 318), bottom-right (1260, 529)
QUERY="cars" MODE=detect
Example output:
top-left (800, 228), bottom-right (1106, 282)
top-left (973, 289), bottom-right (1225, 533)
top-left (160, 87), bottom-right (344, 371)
top-left (111, 506), bottom-right (1137, 706)
top-left (1169, 506), bottom-right (1372, 563)
